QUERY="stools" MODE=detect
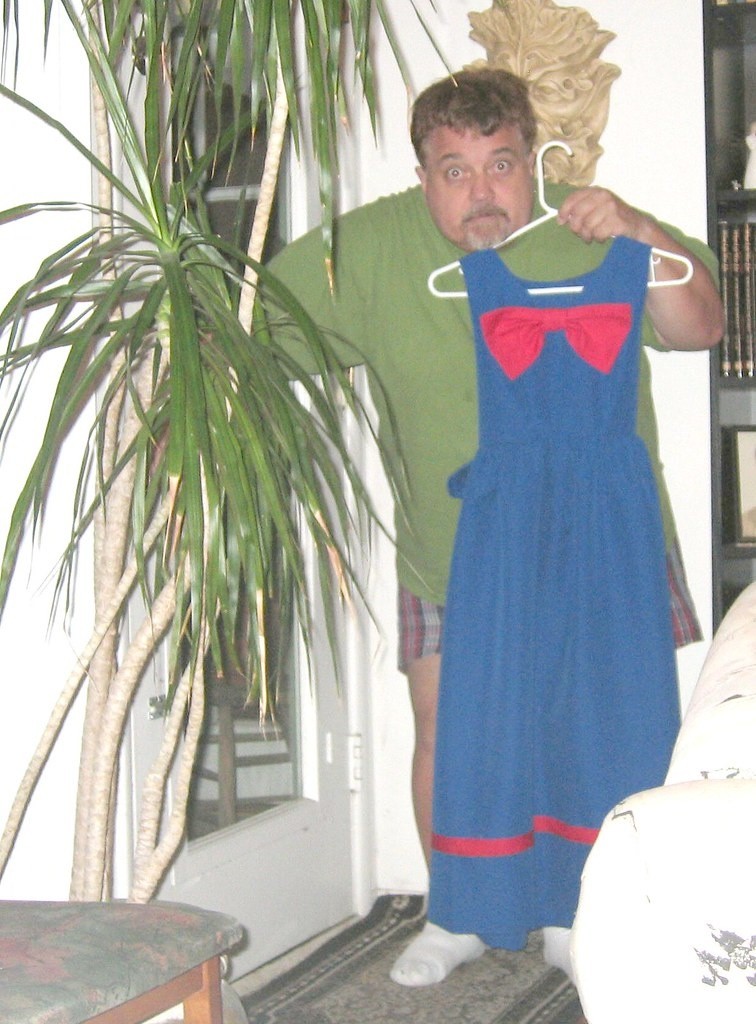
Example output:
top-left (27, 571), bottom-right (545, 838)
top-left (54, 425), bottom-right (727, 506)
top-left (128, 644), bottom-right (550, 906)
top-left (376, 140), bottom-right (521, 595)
top-left (0, 899), bottom-right (245, 1023)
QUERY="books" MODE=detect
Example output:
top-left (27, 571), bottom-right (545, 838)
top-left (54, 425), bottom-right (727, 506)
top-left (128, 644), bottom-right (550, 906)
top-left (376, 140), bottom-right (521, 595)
top-left (717, 221), bottom-right (756, 378)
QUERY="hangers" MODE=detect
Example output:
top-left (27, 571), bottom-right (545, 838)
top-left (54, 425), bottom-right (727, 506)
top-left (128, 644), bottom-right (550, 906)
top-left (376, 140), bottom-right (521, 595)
top-left (427, 140), bottom-right (693, 300)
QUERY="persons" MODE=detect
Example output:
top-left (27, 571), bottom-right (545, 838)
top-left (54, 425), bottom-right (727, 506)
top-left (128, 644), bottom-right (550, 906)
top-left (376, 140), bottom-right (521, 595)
top-left (260, 70), bottom-right (727, 986)
